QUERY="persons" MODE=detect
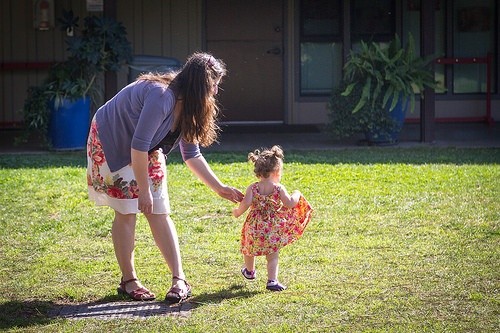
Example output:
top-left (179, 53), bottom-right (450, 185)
top-left (231, 143), bottom-right (313, 292)
top-left (85, 52), bottom-right (246, 306)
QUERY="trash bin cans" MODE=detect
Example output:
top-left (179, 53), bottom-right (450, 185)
top-left (127, 52), bottom-right (182, 87)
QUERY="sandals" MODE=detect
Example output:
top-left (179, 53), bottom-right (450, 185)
top-left (166, 276), bottom-right (191, 301)
top-left (266, 281), bottom-right (284, 290)
top-left (241, 268), bottom-right (255, 279)
top-left (118, 277), bottom-right (156, 301)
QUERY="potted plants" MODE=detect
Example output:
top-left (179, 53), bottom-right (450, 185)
top-left (341, 32), bottom-right (432, 147)
top-left (23, 6), bottom-right (128, 152)
